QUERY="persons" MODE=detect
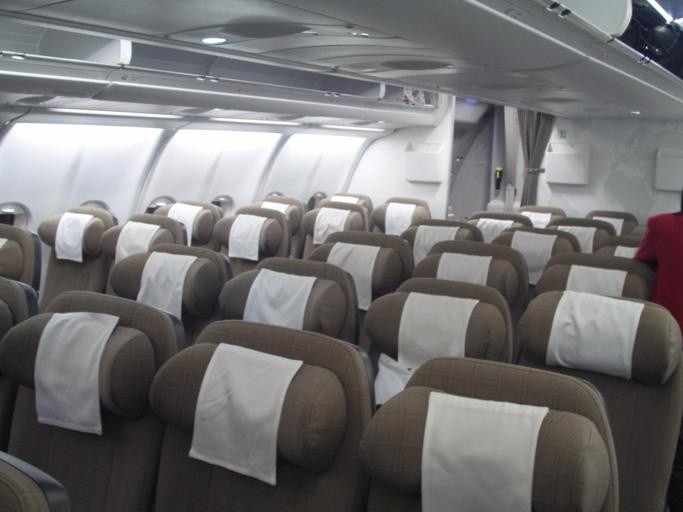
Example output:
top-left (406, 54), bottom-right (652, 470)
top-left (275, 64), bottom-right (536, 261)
top-left (630, 188), bottom-right (682, 512)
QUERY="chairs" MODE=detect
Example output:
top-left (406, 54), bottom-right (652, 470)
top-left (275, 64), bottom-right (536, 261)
top-left (0, 184), bottom-right (374, 384)
top-left (357, 352), bottom-right (624, 511)
top-left (0, 285), bottom-right (182, 511)
top-left (513, 280), bottom-right (682, 512)
top-left (138, 316), bottom-right (376, 512)
top-left (1, 439), bottom-right (72, 512)
top-left (374, 190), bottom-right (662, 384)
